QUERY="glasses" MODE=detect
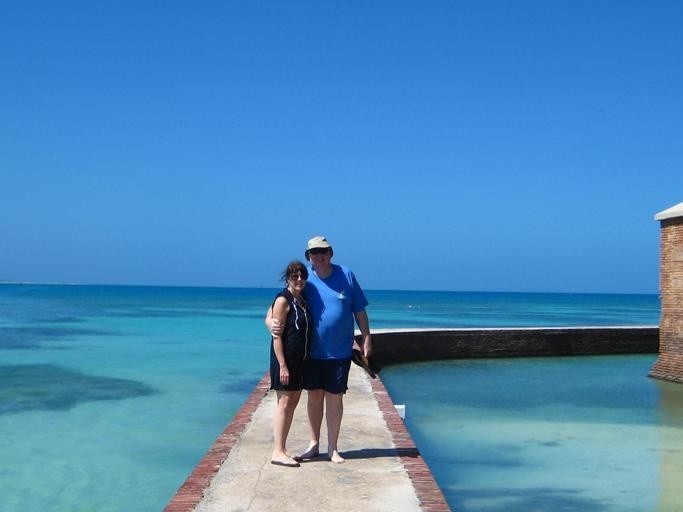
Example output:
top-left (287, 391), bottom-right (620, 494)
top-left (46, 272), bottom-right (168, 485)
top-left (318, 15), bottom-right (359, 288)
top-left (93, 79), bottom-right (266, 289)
top-left (307, 248), bottom-right (328, 254)
top-left (291, 273), bottom-right (305, 280)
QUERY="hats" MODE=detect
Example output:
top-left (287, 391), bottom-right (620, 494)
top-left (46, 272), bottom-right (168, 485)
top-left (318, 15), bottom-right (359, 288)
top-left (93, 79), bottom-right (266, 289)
top-left (305, 236), bottom-right (333, 261)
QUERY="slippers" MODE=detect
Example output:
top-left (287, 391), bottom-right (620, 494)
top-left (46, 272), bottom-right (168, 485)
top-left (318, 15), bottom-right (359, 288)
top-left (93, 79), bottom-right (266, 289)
top-left (271, 457), bottom-right (303, 466)
top-left (352, 349), bottom-right (381, 378)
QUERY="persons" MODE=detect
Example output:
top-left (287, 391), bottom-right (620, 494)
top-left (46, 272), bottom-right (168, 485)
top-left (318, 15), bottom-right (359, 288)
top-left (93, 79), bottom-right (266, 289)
top-left (271, 261), bottom-right (312, 467)
top-left (265, 235), bottom-right (372, 463)
